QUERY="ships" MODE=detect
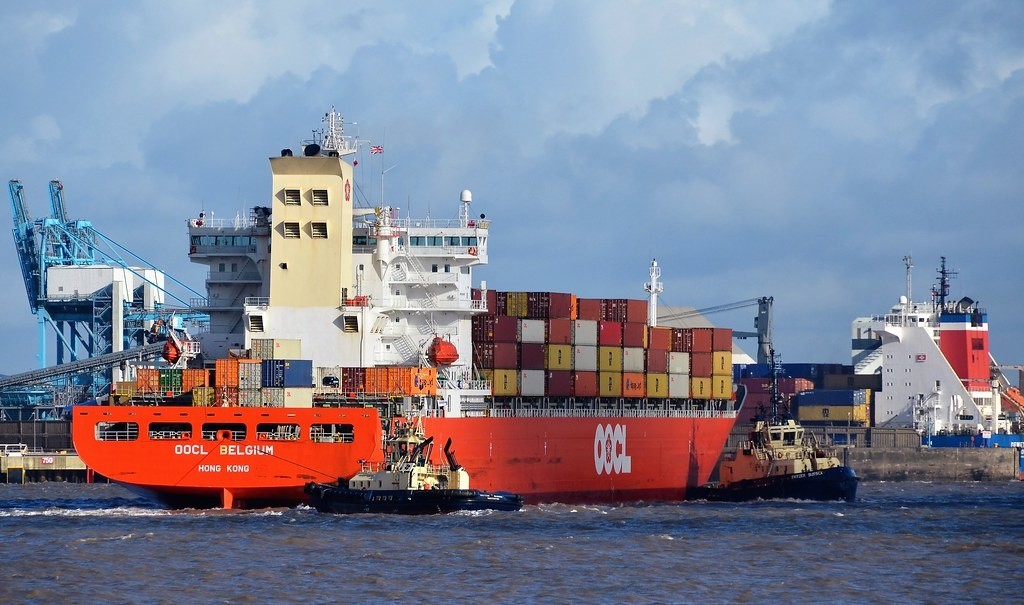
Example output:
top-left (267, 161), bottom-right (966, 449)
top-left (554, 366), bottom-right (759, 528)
top-left (72, 102), bottom-right (748, 510)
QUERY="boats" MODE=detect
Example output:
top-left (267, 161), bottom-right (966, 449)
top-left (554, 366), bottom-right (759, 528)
top-left (704, 417), bottom-right (861, 502)
top-left (303, 413), bottom-right (525, 515)
